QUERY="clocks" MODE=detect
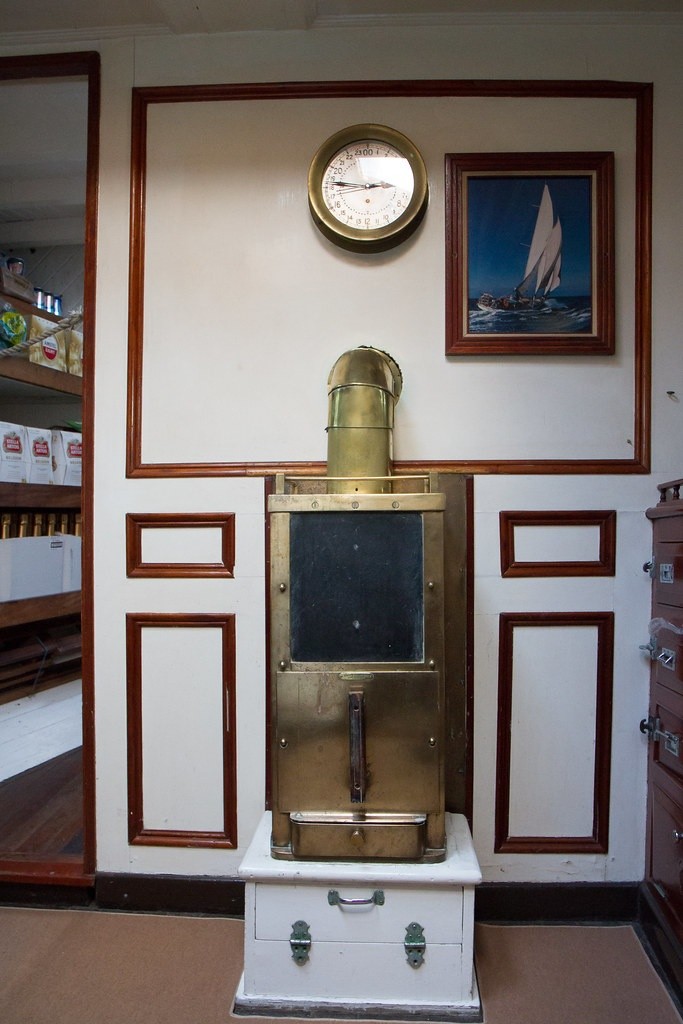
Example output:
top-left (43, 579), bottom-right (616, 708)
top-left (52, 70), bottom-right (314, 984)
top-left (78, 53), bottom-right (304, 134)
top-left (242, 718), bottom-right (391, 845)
top-left (308, 122), bottom-right (429, 239)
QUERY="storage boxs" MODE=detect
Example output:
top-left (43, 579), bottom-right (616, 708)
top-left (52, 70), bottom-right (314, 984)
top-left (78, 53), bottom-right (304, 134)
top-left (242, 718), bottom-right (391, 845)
top-left (0, 267), bottom-right (37, 304)
top-left (23, 313), bottom-right (69, 373)
top-left (63, 330), bottom-right (84, 376)
top-left (0, 421), bottom-right (32, 483)
top-left (56, 530), bottom-right (81, 592)
top-left (26, 426), bottom-right (53, 484)
top-left (0, 533), bottom-right (64, 605)
top-left (51, 429), bottom-right (82, 486)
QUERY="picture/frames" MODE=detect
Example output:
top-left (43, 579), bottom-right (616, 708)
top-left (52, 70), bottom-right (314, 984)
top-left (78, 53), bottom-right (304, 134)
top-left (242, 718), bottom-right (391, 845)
top-left (121, 78), bottom-right (655, 477)
top-left (125, 613), bottom-right (240, 850)
top-left (442, 151), bottom-right (618, 357)
top-left (126, 512), bottom-right (236, 580)
top-left (494, 611), bottom-right (615, 855)
top-left (496, 509), bottom-right (618, 578)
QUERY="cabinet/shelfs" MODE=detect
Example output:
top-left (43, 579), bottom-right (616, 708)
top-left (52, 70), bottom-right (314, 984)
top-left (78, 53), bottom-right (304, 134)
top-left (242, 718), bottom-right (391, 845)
top-left (0, 294), bottom-right (83, 785)
top-left (639, 478), bottom-right (683, 1001)
top-left (238, 811), bottom-right (484, 1008)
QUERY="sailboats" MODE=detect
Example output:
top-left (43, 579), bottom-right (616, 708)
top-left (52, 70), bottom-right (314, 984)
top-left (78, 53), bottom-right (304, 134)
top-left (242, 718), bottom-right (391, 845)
top-left (477, 183), bottom-right (568, 315)
